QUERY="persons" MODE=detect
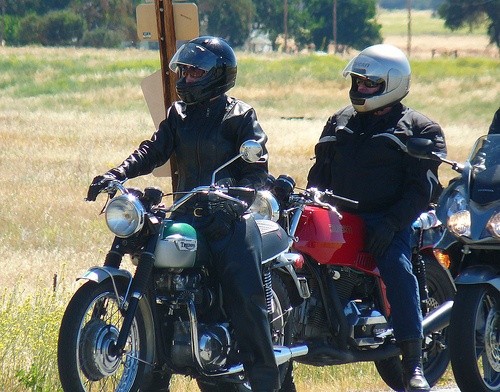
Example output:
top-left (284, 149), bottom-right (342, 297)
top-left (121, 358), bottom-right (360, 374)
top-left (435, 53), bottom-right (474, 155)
top-left (88, 36), bottom-right (281, 392)
top-left (470, 107), bottom-right (500, 166)
top-left (305, 43), bottom-right (448, 392)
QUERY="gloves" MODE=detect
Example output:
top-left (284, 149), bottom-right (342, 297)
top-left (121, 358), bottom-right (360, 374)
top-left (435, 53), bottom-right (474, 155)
top-left (366, 217), bottom-right (400, 259)
top-left (86, 169), bottom-right (126, 201)
top-left (198, 210), bottom-right (232, 241)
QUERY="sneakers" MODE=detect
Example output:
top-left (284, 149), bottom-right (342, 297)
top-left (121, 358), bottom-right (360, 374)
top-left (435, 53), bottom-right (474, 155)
top-left (401, 347), bottom-right (430, 392)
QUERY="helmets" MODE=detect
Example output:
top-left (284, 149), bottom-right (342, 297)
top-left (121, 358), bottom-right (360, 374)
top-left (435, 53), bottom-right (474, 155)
top-left (349, 43), bottom-right (411, 113)
top-left (176, 36), bottom-right (237, 105)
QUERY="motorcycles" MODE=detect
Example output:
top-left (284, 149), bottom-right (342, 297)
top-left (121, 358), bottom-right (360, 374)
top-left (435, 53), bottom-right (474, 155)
top-left (403, 131), bottom-right (500, 392)
top-left (56, 140), bottom-right (312, 392)
top-left (265, 172), bottom-right (458, 392)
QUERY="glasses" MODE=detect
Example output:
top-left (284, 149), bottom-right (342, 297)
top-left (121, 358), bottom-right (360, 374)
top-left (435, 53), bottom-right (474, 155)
top-left (181, 68), bottom-right (205, 78)
top-left (355, 77), bottom-right (378, 88)
